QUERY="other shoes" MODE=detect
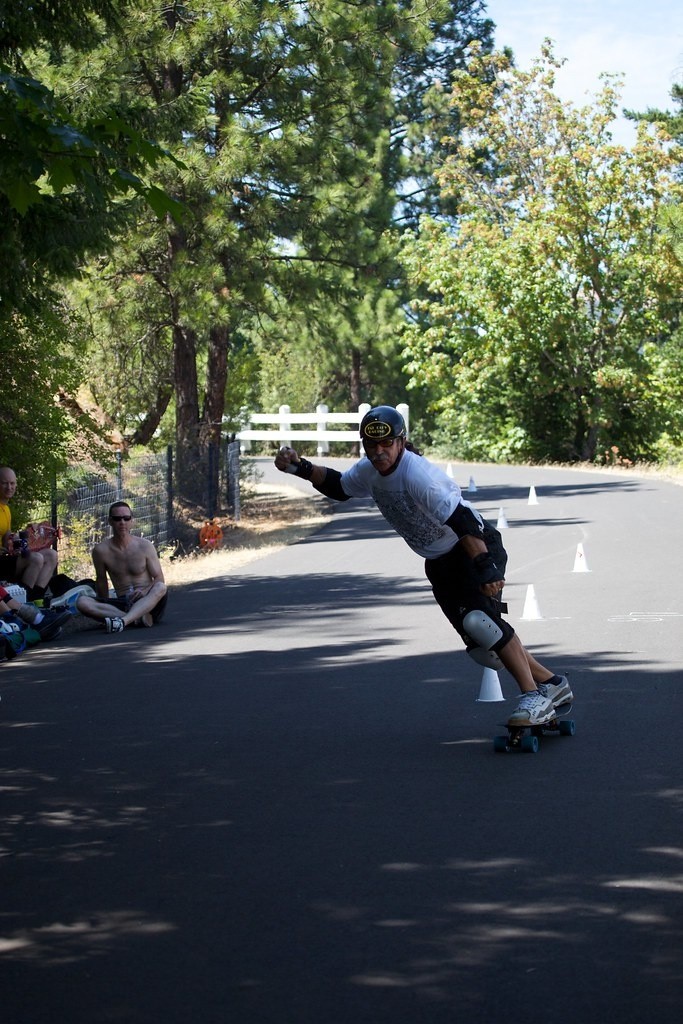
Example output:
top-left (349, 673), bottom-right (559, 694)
top-left (31, 608), bottom-right (70, 641)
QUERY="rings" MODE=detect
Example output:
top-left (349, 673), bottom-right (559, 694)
top-left (499, 588), bottom-right (501, 591)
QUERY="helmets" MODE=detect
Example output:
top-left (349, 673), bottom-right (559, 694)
top-left (360, 406), bottom-right (406, 437)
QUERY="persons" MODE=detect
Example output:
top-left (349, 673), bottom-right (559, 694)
top-left (76, 502), bottom-right (168, 633)
top-left (0, 584), bottom-right (72, 643)
top-left (0, 467), bottom-right (58, 608)
top-left (274, 406), bottom-right (574, 727)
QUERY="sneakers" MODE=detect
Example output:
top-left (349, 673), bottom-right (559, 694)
top-left (509, 688), bottom-right (556, 725)
top-left (538, 675), bottom-right (574, 707)
top-left (134, 613), bottom-right (154, 626)
top-left (105, 617), bottom-right (124, 632)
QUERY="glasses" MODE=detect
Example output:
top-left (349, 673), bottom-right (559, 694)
top-left (363, 437), bottom-right (398, 448)
top-left (111, 515), bottom-right (131, 522)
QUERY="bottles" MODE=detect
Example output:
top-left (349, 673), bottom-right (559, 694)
top-left (38, 528), bottom-right (46, 545)
top-left (126, 585), bottom-right (135, 610)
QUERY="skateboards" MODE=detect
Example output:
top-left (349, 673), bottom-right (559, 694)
top-left (4, 521), bottom-right (61, 557)
top-left (491, 700), bottom-right (577, 755)
